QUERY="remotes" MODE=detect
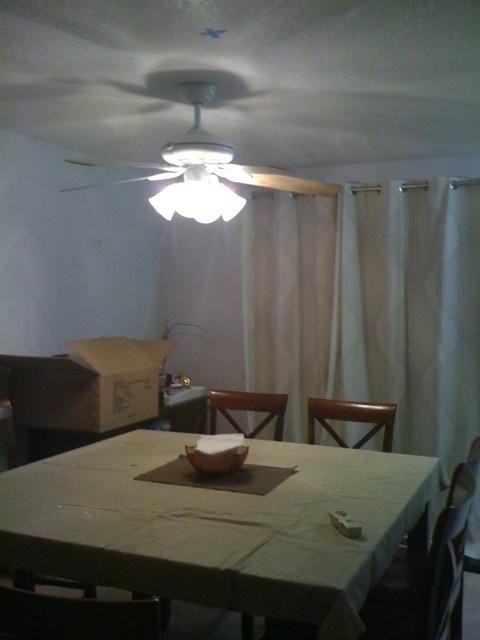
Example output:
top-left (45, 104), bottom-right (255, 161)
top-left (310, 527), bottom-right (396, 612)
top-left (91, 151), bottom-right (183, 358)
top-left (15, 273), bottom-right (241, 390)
top-left (329, 510), bottom-right (362, 538)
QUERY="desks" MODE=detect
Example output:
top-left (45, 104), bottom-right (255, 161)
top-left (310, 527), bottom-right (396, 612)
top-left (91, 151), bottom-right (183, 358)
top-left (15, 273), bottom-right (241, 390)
top-left (1, 429), bottom-right (450, 640)
top-left (28, 385), bottom-right (210, 459)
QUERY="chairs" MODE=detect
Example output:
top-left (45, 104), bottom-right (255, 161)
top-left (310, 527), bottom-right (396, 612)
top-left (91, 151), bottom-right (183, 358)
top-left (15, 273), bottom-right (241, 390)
top-left (266, 436), bottom-right (480, 640)
top-left (1, 583), bottom-right (163, 640)
top-left (205, 388), bottom-right (289, 441)
top-left (308, 397), bottom-right (396, 451)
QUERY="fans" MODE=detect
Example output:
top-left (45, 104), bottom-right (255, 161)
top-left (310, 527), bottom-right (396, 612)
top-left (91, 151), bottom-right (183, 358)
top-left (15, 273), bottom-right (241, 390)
top-left (57, 81), bottom-right (344, 197)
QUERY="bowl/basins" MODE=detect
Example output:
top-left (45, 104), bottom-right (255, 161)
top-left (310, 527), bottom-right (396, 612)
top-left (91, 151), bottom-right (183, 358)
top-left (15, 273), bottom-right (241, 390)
top-left (184, 443), bottom-right (249, 473)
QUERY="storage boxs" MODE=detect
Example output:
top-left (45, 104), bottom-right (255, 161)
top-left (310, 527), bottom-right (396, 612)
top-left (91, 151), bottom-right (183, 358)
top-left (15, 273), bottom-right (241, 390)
top-left (1, 335), bottom-right (178, 434)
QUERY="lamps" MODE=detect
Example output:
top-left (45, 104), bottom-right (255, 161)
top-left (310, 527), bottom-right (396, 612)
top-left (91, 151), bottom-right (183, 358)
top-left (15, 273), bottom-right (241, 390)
top-left (148, 166), bottom-right (247, 225)
